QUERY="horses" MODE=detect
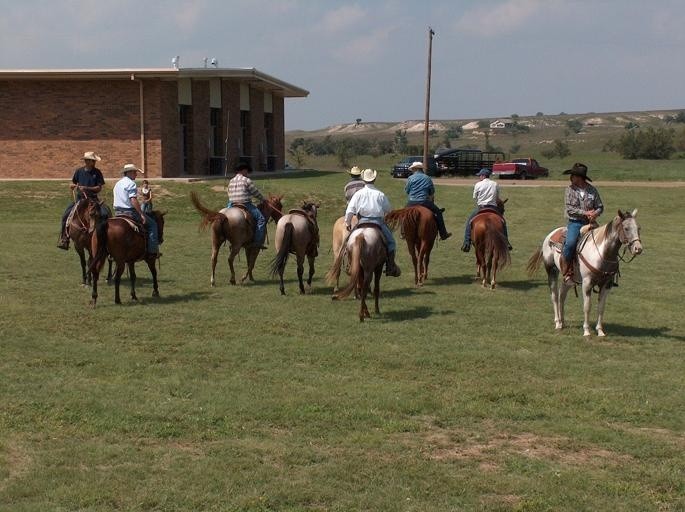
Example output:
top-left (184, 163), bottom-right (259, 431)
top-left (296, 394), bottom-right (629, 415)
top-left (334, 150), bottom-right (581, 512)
top-left (323, 214), bottom-right (362, 300)
top-left (189, 190), bottom-right (284, 287)
top-left (383, 193), bottom-right (439, 289)
top-left (264, 201), bottom-right (323, 295)
top-left (332, 226), bottom-right (386, 323)
top-left (69, 197), bottom-right (114, 292)
top-left (470, 197), bottom-right (511, 292)
top-left (88, 206), bottom-right (168, 307)
top-left (525, 208), bottom-right (644, 338)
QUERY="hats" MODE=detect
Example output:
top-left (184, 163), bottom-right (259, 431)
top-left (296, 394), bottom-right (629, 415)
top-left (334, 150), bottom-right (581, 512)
top-left (476, 169), bottom-right (492, 177)
top-left (407, 162), bottom-right (428, 171)
top-left (346, 167), bottom-right (364, 176)
top-left (563, 162), bottom-right (593, 182)
top-left (361, 169), bottom-right (377, 182)
top-left (79, 151), bottom-right (102, 162)
top-left (232, 160), bottom-right (253, 172)
top-left (121, 163), bottom-right (145, 174)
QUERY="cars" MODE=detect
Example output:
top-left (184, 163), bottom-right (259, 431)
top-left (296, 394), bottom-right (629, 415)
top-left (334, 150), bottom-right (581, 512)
top-left (392, 155), bottom-right (442, 178)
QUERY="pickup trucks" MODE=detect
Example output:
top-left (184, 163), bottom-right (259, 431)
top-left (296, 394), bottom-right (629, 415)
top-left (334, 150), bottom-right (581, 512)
top-left (492, 158), bottom-right (548, 179)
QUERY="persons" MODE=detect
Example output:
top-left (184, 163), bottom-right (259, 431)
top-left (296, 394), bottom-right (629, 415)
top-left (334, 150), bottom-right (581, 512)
top-left (344, 166), bottom-right (365, 214)
top-left (56, 151), bottom-right (105, 250)
top-left (344, 168), bottom-right (401, 277)
top-left (460, 168), bottom-right (512, 251)
top-left (227, 162), bottom-right (266, 252)
top-left (560, 163), bottom-right (604, 287)
top-left (141, 180), bottom-right (152, 213)
top-left (399, 162), bottom-right (452, 240)
top-left (113, 163), bottom-right (158, 260)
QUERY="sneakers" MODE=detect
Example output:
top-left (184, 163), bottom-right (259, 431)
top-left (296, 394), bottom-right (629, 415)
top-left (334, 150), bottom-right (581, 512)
top-left (442, 232), bottom-right (452, 240)
top-left (57, 241), bottom-right (69, 249)
top-left (400, 233), bottom-right (404, 240)
top-left (508, 243), bottom-right (513, 251)
top-left (146, 252), bottom-right (163, 260)
top-left (252, 242), bottom-right (268, 249)
top-left (460, 245), bottom-right (470, 252)
top-left (564, 277), bottom-right (579, 287)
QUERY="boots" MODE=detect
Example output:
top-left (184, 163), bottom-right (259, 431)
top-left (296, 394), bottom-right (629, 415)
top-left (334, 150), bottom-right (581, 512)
top-left (385, 250), bottom-right (400, 276)
